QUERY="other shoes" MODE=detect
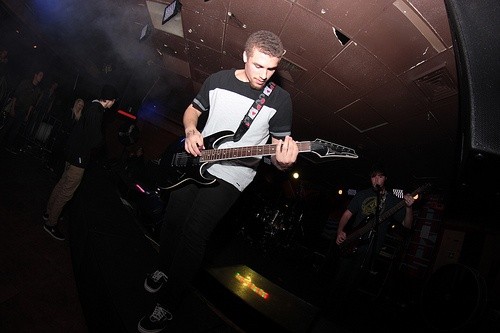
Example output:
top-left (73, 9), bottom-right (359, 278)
top-left (6, 146), bottom-right (17, 154)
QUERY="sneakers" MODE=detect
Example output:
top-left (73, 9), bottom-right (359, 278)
top-left (43, 224), bottom-right (66, 240)
top-left (43, 213), bottom-right (64, 221)
top-left (138, 306), bottom-right (171, 333)
top-left (144, 269), bottom-right (165, 293)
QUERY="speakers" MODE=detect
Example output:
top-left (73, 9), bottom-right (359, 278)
top-left (444, 0), bottom-right (500, 162)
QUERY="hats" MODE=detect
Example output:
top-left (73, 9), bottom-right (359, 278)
top-left (101, 84), bottom-right (118, 99)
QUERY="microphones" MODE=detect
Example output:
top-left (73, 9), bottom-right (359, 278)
top-left (376, 184), bottom-right (383, 194)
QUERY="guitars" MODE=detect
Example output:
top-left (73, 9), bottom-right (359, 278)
top-left (343, 188), bottom-right (424, 243)
top-left (154, 131), bottom-right (359, 190)
